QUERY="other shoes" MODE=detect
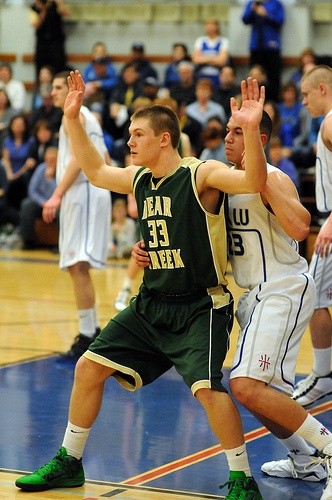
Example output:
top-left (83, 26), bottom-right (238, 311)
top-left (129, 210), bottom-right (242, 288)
top-left (114, 287), bottom-right (131, 310)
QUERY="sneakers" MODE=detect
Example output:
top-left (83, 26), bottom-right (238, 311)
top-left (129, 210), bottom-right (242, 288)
top-left (219, 471), bottom-right (262, 500)
top-left (260, 451), bottom-right (327, 482)
top-left (66, 329), bottom-right (101, 356)
top-left (290, 370), bottom-right (332, 407)
top-left (320, 443), bottom-right (332, 500)
top-left (16, 445), bottom-right (85, 489)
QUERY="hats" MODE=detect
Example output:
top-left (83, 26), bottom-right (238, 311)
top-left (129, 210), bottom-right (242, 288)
top-left (132, 45), bottom-right (143, 51)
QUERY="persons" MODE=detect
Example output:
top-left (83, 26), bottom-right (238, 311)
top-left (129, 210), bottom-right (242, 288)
top-left (41, 72), bottom-right (112, 360)
top-left (242, 0), bottom-right (284, 102)
top-left (131, 109), bottom-right (332, 500)
top-left (288, 65), bottom-right (332, 407)
top-left (178, 17), bottom-right (235, 90)
top-left (14, 70), bottom-right (267, 500)
top-left (27, 0), bottom-right (72, 85)
top-left (0, 40), bottom-right (332, 259)
